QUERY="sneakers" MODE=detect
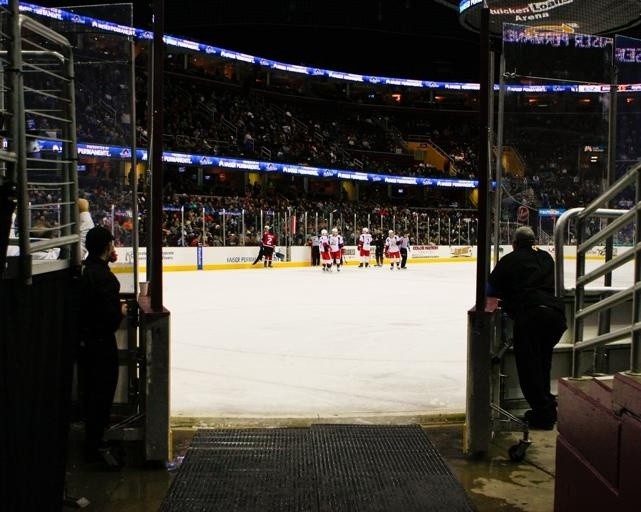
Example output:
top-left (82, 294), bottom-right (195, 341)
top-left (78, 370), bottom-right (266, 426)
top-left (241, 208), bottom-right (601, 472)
top-left (83, 433), bottom-right (115, 458)
top-left (523, 405), bottom-right (558, 431)
top-left (321, 264), bottom-right (408, 273)
top-left (263, 264), bottom-right (273, 268)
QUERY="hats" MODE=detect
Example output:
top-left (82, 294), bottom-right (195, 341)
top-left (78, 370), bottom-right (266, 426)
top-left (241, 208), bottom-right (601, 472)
top-left (86, 225), bottom-right (118, 253)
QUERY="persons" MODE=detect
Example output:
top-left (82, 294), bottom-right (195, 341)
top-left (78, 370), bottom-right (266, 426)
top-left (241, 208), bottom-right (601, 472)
top-left (28, 184), bottom-right (477, 246)
top-left (329, 227), bottom-right (345, 271)
top-left (253, 224), bottom-right (270, 265)
top-left (488, 225), bottom-right (571, 430)
top-left (76, 197), bottom-right (98, 262)
top-left (398, 233), bottom-right (412, 270)
top-left (357, 226), bottom-right (373, 268)
top-left (307, 231), bottom-right (320, 266)
top-left (385, 229), bottom-right (402, 271)
top-left (69, 227), bottom-right (129, 465)
top-left (500, 115), bottom-right (641, 246)
top-left (0, 51), bottom-right (479, 180)
top-left (318, 228), bottom-right (333, 272)
top-left (260, 226), bottom-right (277, 267)
top-left (370, 234), bottom-right (385, 267)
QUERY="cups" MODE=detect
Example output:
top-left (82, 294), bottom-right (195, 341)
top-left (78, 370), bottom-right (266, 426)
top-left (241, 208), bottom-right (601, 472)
top-left (138, 281), bottom-right (150, 297)
top-left (109, 248), bottom-right (117, 263)
